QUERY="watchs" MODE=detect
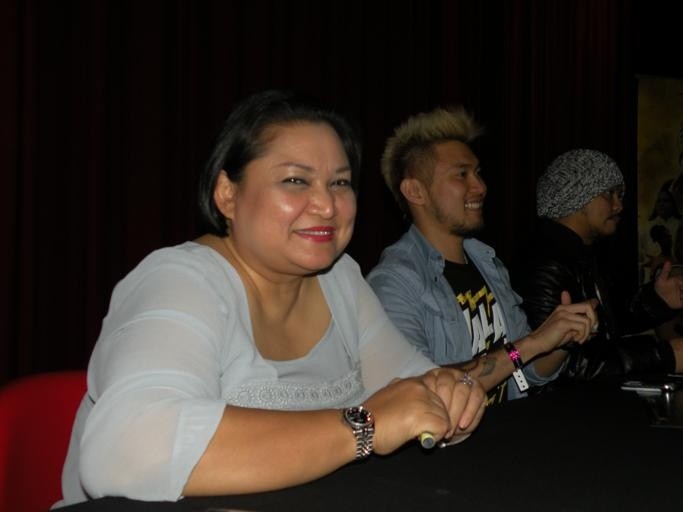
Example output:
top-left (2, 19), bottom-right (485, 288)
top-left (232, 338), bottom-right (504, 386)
top-left (340, 402), bottom-right (376, 459)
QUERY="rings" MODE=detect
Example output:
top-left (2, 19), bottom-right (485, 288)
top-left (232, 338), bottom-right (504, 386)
top-left (456, 375), bottom-right (474, 387)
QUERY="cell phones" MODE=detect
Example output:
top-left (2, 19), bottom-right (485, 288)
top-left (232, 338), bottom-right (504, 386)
top-left (620, 379), bottom-right (675, 393)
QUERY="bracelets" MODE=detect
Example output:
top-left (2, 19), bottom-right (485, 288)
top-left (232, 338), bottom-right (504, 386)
top-left (503, 341), bottom-right (530, 392)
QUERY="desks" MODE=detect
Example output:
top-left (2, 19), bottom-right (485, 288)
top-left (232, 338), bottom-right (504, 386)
top-left (50, 376), bottom-right (682, 512)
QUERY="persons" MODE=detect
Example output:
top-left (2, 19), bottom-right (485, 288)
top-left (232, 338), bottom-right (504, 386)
top-left (45, 90), bottom-right (487, 511)
top-left (508, 146), bottom-right (682, 397)
top-left (363, 102), bottom-right (602, 405)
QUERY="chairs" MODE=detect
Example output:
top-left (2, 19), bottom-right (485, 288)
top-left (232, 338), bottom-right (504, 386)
top-left (1, 371), bottom-right (90, 511)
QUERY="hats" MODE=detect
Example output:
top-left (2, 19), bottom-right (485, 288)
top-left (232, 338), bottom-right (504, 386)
top-left (535, 148), bottom-right (623, 219)
